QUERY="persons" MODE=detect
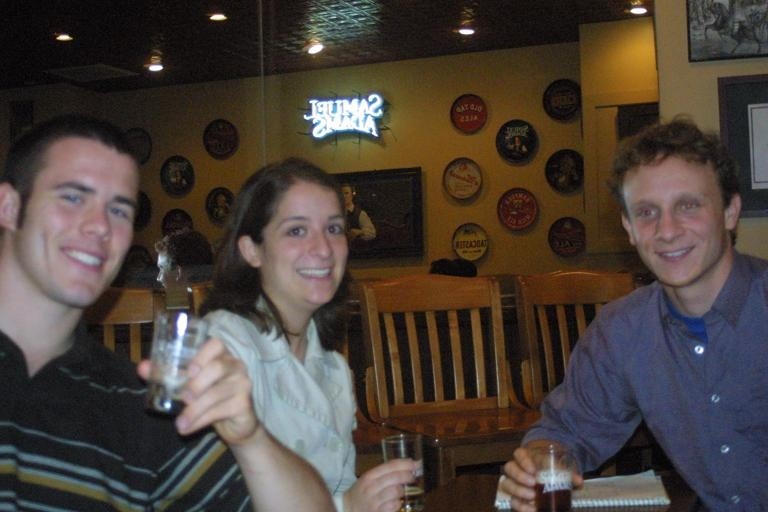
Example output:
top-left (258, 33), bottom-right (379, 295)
top-left (348, 259), bottom-right (526, 399)
top-left (502, 115), bottom-right (767, 511)
top-left (1, 113), bottom-right (336, 512)
top-left (335, 184), bottom-right (377, 256)
top-left (185, 155), bottom-right (417, 511)
top-left (118, 234), bottom-right (216, 345)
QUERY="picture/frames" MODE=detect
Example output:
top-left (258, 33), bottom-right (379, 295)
top-left (685, 1), bottom-right (768, 218)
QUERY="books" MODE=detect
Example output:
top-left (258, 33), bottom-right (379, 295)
top-left (494, 468), bottom-right (669, 507)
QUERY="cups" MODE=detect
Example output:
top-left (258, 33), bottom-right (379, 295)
top-left (142, 309), bottom-right (209, 417)
top-left (380, 434), bottom-right (425, 512)
top-left (528, 446), bottom-right (570, 512)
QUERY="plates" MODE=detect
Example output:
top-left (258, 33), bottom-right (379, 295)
top-left (442, 78), bottom-right (587, 263)
top-left (121, 117), bottom-right (240, 279)
